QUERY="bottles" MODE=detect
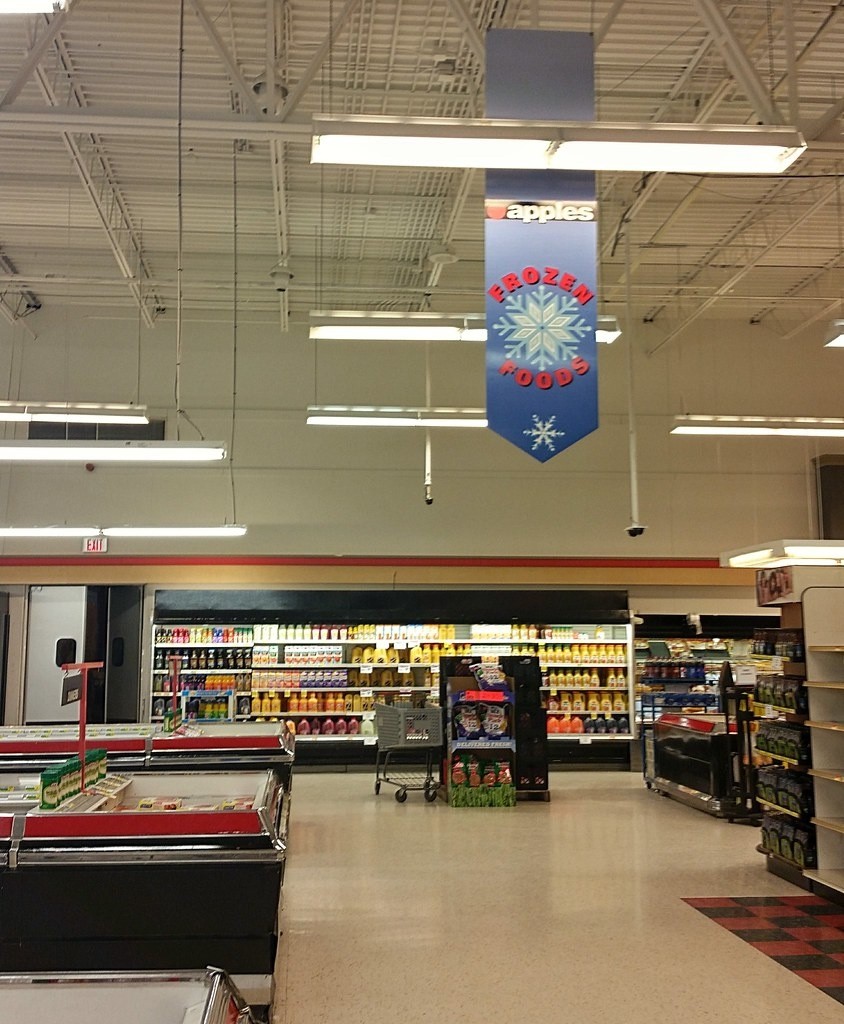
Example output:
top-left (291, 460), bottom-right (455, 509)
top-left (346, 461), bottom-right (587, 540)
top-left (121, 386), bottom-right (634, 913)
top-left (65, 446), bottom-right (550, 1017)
top-left (151, 622), bottom-right (629, 734)
top-left (39, 747), bottom-right (107, 808)
top-left (643, 655), bottom-right (705, 679)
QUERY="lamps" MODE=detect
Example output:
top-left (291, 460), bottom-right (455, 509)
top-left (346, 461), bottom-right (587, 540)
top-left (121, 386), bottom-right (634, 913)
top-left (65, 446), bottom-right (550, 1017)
top-left (0, 0), bottom-right (844, 538)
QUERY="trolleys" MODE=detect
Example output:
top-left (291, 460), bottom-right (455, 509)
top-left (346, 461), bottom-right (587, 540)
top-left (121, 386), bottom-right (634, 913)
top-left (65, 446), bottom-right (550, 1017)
top-left (375, 700), bottom-right (443, 803)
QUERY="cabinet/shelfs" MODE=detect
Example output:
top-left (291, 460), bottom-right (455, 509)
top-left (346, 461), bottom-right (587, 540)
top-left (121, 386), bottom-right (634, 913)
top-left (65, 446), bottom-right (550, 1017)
top-left (447, 676), bottom-right (516, 808)
top-left (635, 585), bottom-right (844, 909)
